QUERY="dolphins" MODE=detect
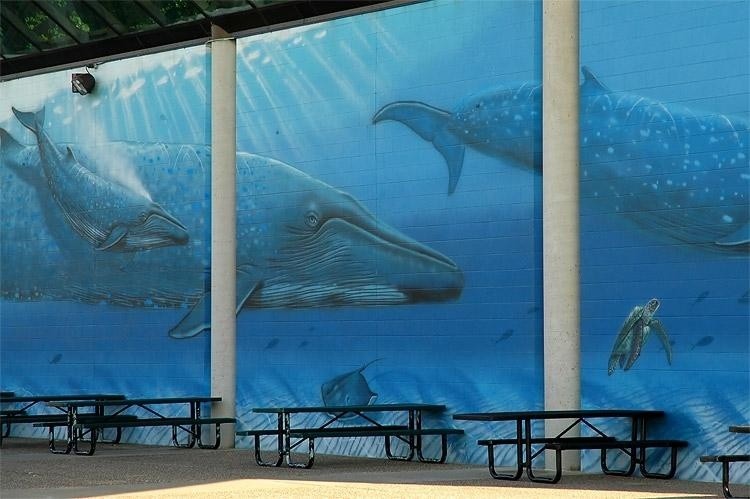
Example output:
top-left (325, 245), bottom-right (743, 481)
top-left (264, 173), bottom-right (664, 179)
top-left (10, 106), bottom-right (190, 253)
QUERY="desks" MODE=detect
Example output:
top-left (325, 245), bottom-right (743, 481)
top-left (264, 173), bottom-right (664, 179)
top-left (253, 403), bottom-right (445, 469)
top-left (728, 426), bottom-right (750, 433)
top-left (453, 410), bottom-right (664, 482)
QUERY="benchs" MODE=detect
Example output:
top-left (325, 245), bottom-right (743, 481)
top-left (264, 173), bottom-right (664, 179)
top-left (236, 426), bottom-right (408, 466)
top-left (700, 454), bottom-right (750, 498)
top-left (1, 409), bottom-right (236, 456)
top-left (533, 440), bottom-right (689, 483)
top-left (478, 437), bottom-right (618, 480)
top-left (291, 428), bottom-right (464, 468)
